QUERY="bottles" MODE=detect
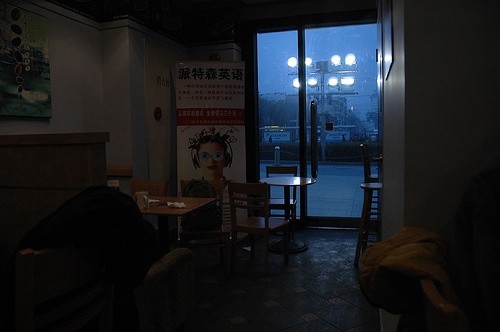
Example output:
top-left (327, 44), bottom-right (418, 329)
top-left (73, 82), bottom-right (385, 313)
top-left (275, 146), bottom-right (281, 166)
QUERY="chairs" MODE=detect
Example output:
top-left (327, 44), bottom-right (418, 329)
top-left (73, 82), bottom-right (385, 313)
top-left (179, 179), bottom-right (227, 269)
top-left (227, 182), bottom-right (291, 276)
top-left (134, 177), bottom-right (167, 196)
top-left (14, 241), bottom-right (118, 332)
top-left (267, 165), bottom-right (297, 239)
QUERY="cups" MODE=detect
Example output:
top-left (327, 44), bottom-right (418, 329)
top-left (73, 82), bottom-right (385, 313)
top-left (135, 191), bottom-right (149, 209)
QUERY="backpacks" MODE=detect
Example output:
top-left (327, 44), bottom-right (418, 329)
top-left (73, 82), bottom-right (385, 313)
top-left (181, 180), bottom-right (223, 231)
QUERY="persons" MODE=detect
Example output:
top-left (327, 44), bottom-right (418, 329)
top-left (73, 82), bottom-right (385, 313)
top-left (196, 137), bottom-right (244, 202)
top-left (449, 168), bottom-right (500, 332)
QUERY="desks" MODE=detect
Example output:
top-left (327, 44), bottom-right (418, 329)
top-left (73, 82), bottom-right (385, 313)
top-left (131, 196), bottom-right (219, 251)
top-left (259, 176), bottom-right (315, 254)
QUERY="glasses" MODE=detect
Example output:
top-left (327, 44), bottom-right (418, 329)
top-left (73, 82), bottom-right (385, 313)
top-left (199, 152), bottom-right (226, 161)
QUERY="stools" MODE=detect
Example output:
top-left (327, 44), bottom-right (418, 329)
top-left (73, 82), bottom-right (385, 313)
top-left (355, 143), bottom-right (385, 266)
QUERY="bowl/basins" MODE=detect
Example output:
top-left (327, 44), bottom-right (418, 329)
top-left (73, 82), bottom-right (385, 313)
top-left (146, 199), bottom-right (160, 207)
